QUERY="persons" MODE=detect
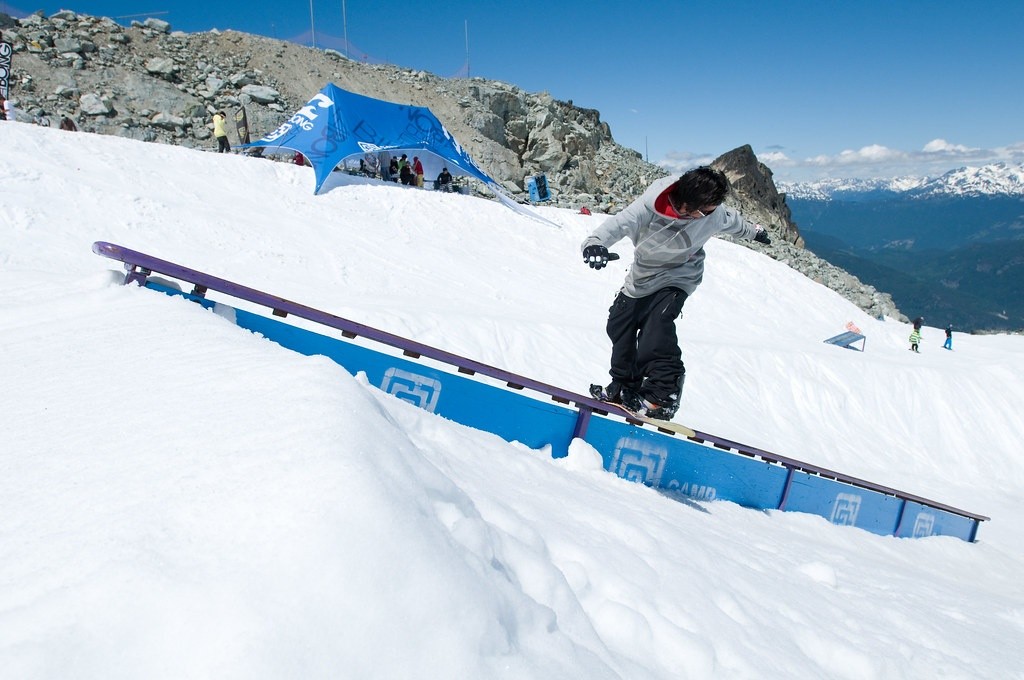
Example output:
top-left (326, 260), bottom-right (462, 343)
top-left (944, 324), bottom-right (953, 349)
top-left (912, 317), bottom-right (924, 332)
top-left (213, 111), bottom-right (230, 154)
top-left (389, 154), bottom-right (424, 187)
top-left (58, 113), bottom-right (76, 132)
top-left (583, 165), bottom-right (772, 421)
top-left (0, 93), bottom-right (16, 121)
top-left (909, 329), bottom-right (920, 352)
top-left (437, 168), bottom-right (453, 193)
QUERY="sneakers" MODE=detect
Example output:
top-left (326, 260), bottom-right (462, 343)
top-left (621, 374), bottom-right (684, 420)
top-left (589, 373), bottom-right (643, 404)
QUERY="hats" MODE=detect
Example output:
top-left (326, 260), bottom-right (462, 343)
top-left (442, 168), bottom-right (448, 171)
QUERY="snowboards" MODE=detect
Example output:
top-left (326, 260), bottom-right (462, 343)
top-left (590, 384), bottom-right (696, 438)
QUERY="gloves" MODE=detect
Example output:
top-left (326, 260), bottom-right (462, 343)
top-left (754, 229), bottom-right (771, 245)
top-left (582, 244), bottom-right (619, 271)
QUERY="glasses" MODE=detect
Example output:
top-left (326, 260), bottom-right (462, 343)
top-left (696, 206), bottom-right (717, 217)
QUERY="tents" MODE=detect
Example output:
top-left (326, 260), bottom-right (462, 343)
top-left (207, 83), bottom-right (562, 229)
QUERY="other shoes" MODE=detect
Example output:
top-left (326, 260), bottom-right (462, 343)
top-left (948, 348), bottom-right (953, 350)
top-left (942, 345), bottom-right (947, 349)
top-left (908, 348), bottom-right (914, 351)
top-left (915, 350), bottom-right (919, 353)
